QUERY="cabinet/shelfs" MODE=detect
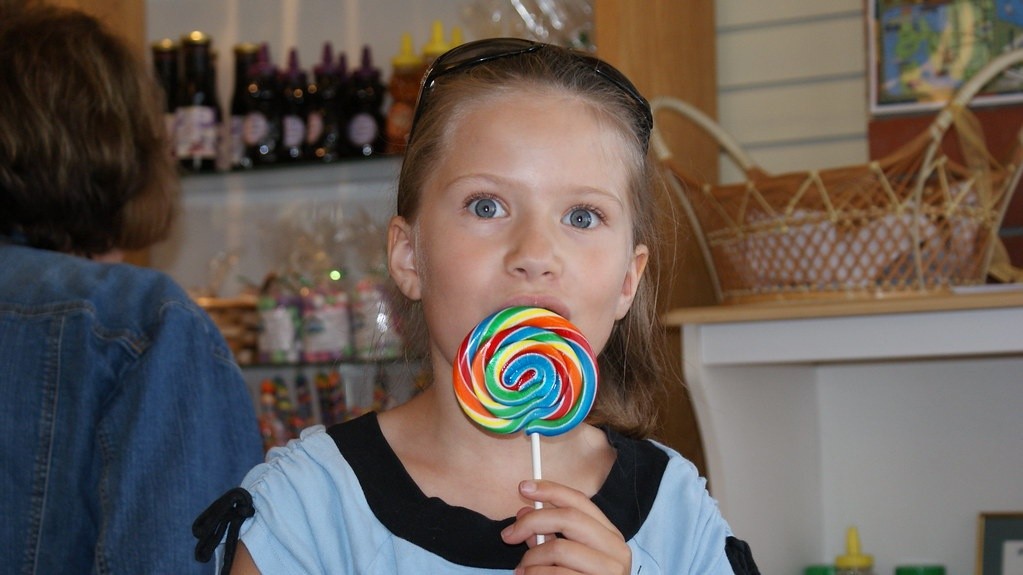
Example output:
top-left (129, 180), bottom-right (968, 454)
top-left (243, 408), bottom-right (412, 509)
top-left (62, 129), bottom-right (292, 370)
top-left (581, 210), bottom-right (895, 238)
top-left (659, 282), bottom-right (1023, 575)
top-left (25, 1), bottom-right (721, 494)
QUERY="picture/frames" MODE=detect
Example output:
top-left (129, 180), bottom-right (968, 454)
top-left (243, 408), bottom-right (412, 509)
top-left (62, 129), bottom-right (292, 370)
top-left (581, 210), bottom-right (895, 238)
top-left (977, 510), bottom-right (1023, 575)
top-left (866, 1), bottom-right (1023, 115)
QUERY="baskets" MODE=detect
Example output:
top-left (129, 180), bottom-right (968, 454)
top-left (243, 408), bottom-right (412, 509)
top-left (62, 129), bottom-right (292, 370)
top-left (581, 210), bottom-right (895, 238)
top-left (645, 55), bottom-right (1022, 304)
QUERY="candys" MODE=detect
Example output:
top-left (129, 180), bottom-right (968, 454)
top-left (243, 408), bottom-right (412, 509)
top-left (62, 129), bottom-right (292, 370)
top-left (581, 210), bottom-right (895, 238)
top-left (451, 305), bottom-right (599, 544)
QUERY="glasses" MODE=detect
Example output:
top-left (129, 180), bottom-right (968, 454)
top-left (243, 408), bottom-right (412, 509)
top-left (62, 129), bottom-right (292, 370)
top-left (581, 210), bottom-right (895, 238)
top-left (395, 37), bottom-right (654, 208)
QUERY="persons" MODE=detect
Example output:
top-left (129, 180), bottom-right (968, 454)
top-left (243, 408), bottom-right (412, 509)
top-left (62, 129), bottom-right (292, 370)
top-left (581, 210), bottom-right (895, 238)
top-left (0, 0), bottom-right (263, 573)
top-left (204, 36), bottom-right (739, 575)
